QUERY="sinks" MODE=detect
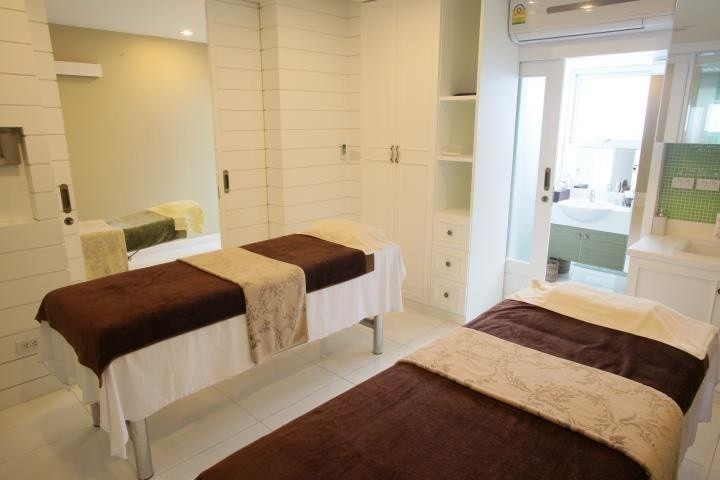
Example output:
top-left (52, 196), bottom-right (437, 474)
top-left (670, 231), bottom-right (720, 271)
top-left (557, 199), bottom-right (615, 222)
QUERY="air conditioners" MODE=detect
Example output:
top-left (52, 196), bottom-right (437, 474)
top-left (509, 1), bottom-right (678, 47)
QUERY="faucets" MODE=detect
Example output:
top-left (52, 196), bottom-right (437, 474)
top-left (589, 189), bottom-right (596, 202)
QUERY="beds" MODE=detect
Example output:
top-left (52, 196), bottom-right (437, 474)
top-left (195, 277), bottom-right (719, 480)
top-left (39, 219), bottom-right (401, 479)
top-left (79, 200), bottom-right (204, 269)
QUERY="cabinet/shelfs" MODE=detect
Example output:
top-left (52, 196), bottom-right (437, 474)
top-left (433, 91), bottom-right (477, 317)
top-left (547, 224), bottom-right (627, 269)
top-left (359, 0), bottom-right (437, 305)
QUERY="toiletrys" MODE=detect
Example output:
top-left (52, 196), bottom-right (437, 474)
top-left (652, 207), bottom-right (667, 235)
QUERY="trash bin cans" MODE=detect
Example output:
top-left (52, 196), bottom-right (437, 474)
top-left (545, 260), bottom-right (560, 283)
